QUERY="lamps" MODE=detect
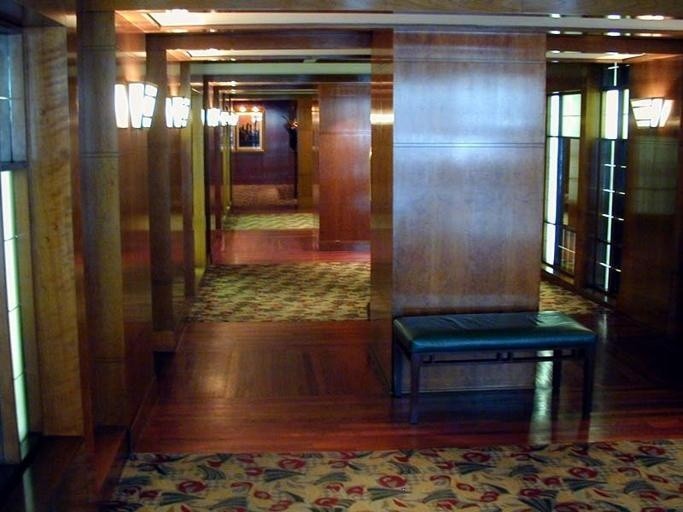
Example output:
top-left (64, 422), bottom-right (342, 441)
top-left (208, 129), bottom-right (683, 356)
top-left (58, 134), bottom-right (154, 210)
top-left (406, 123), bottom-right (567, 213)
top-left (164, 97), bottom-right (191, 129)
top-left (629, 97), bottom-right (674, 129)
top-left (112, 81), bottom-right (157, 129)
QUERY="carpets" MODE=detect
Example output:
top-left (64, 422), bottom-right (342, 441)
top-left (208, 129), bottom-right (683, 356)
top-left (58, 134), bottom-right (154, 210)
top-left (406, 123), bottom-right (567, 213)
top-left (107, 439), bottom-right (683, 512)
top-left (222, 213), bottom-right (319, 230)
top-left (183, 261), bottom-right (606, 321)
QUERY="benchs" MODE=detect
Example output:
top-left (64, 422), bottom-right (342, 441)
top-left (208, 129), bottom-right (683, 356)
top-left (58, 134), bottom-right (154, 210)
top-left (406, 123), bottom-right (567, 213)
top-left (390, 309), bottom-right (598, 425)
